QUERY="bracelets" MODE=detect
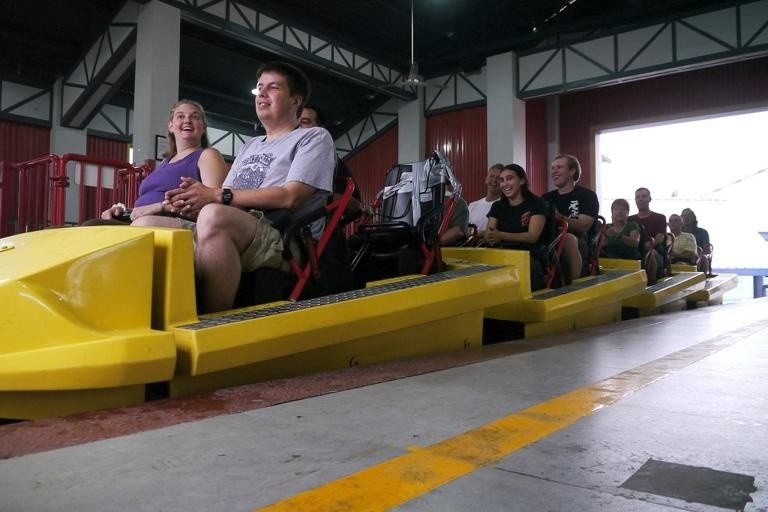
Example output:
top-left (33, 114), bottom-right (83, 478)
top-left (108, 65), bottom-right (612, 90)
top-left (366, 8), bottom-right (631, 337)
top-left (617, 232), bottom-right (623, 240)
top-left (160, 202), bottom-right (165, 215)
top-left (111, 202), bottom-right (127, 210)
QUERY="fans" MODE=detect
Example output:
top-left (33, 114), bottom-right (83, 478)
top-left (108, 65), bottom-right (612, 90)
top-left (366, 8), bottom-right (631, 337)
top-left (374, 0), bottom-right (449, 95)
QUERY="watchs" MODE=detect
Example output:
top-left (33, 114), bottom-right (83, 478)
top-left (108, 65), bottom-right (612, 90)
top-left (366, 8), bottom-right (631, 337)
top-left (221, 189), bottom-right (233, 205)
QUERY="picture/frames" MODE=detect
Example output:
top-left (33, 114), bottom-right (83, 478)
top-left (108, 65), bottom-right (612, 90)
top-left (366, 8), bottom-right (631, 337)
top-left (153, 132), bottom-right (168, 161)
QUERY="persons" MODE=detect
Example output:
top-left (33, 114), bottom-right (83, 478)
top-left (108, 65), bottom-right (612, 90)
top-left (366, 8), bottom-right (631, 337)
top-left (541, 154), bottom-right (599, 281)
top-left (483, 164), bottom-right (552, 292)
top-left (129, 62), bottom-right (338, 313)
top-left (101, 99), bottom-right (228, 222)
top-left (297, 105), bottom-right (361, 227)
top-left (628, 188), bottom-right (666, 286)
top-left (438, 185), bottom-right (470, 246)
top-left (599, 199), bottom-right (642, 258)
top-left (666, 214), bottom-right (698, 264)
top-left (680, 209), bottom-right (709, 273)
top-left (466, 163), bottom-right (504, 240)
